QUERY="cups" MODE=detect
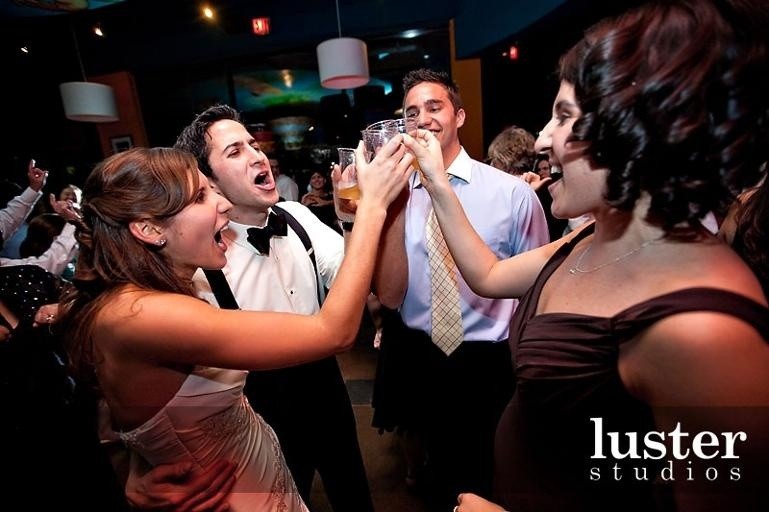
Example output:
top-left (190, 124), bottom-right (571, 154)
top-left (330, 113), bottom-right (420, 224)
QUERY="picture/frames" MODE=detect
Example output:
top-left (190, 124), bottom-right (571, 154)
top-left (109, 134), bottom-right (134, 155)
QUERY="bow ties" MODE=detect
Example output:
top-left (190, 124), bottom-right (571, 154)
top-left (246, 210), bottom-right (288, 257)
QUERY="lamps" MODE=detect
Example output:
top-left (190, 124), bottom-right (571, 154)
top-left (59, 15), bottom-right (119, 123)
top-left (316, 0), bottom-right (371, 90)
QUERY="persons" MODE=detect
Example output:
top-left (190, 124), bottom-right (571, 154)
top-left (0, 0), bottom-right (767, 512)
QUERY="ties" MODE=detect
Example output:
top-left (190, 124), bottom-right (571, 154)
top-left (424, 204), bottom-right (465, 358)
